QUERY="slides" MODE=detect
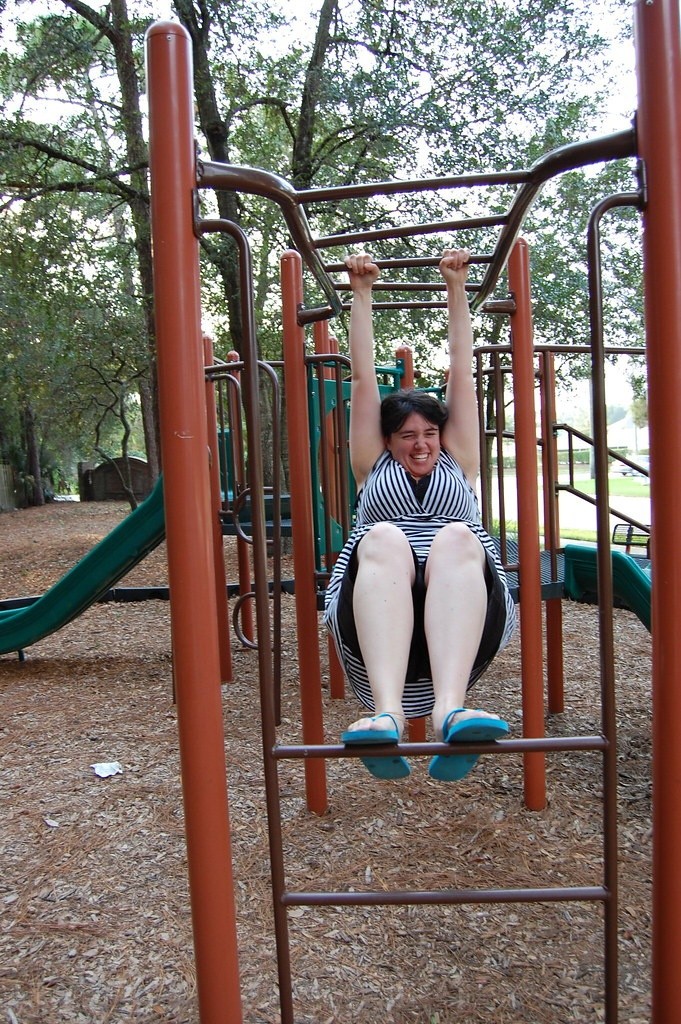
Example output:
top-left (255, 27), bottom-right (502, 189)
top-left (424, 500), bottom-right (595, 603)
top-left (0, 468), bottom-right (165, 657)
top-left (565, 542), bottom-right (655, 636)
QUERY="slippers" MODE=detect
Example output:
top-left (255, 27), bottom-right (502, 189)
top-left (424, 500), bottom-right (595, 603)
top-left (341, 713), bottom-right (412, 780)
top-left (428, 709), bottom-right (511, 781)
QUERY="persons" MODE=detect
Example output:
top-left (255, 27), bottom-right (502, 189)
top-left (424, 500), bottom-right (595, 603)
top-left (322, 246), bottom-right (517, 783)
